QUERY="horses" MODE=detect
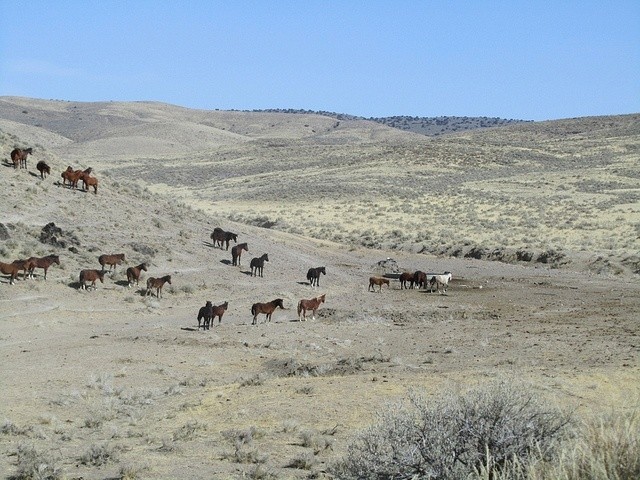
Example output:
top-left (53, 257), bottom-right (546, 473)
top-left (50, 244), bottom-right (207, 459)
top-left (307, 266), bottom-right (326, 287)
top-left (429, 273), bottom-right (452, 293)
top-left (400, 272), bottom-right (415, 289)
top-left (251, 299), bottom-right (283, 324)
top-left (210, 301), bottom-right (228, 327)
top-left (414, 271), bottom-right (428, 289)
top-left (297, 293), bottom-right (326, 321)
top-left (74, 167), bottom-right (93, 191)
top-left (67, 166), bottom-right (74, 173)
top-left (211, 227), bottom-right (237, 250)
top-left (10, 148), bottom-right (33, 170)
top-left (29, 254), bottom-right (60, 281)
top-left (37, 161), bottom-right (50, 178)
top-left (147, 275), bottom-right (172, 298)
top-left (0, 259), bottom-right (32, 285)
top-left (232, 243), bottom-right (248, 266)
top-left (197, 301), bottom-right (213, 330)
top-left (369, 277), bottom-right (389, 292)
top-left (80, 269), bottom-right (104, 291)
top-left (250, 253), bottom-right (269, 278)
top-left (98, 253), bottom-right (128, 270)
top-left (61, 167), bottom-right (83, 190)
top-left (79, 174), bottom-right (98, 192)
top-left (127, 263), bottom-right (147, 284)
